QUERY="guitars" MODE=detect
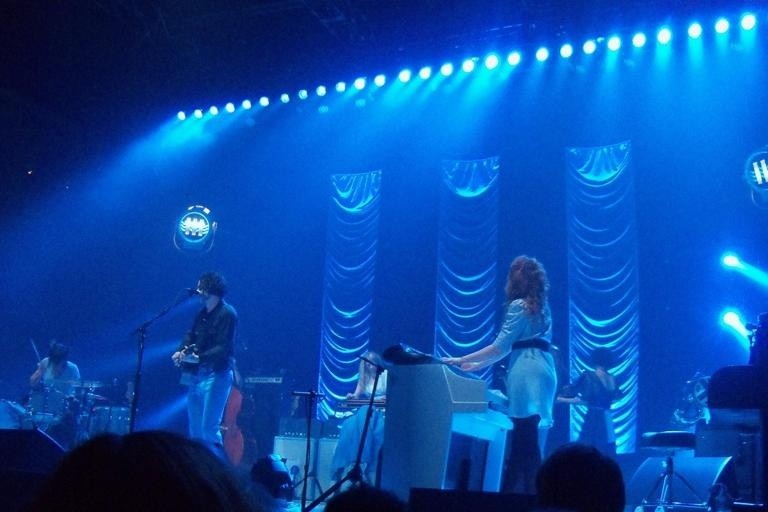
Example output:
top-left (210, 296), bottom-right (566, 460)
top-left (178, 342), bottom-right (200, 385)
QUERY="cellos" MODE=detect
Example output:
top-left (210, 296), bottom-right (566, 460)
top-left (220, 384), bottom-right (258, 474)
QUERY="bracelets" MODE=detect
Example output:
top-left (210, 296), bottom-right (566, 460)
top-left (455, 357), bottom-right (461, 366)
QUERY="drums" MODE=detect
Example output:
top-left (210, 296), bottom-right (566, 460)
top-left (0, 399), bottom-right (131, 449)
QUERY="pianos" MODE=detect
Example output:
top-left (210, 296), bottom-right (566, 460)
top-left (344, 400), bottom-right (387, 407)
top-left (382, 343), bottom-right (484, 380)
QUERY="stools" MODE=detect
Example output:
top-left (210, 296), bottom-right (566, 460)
top-left (634, 430), bottom-right (710, 511)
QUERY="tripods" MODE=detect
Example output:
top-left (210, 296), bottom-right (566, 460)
top-left (305, 356), bottom-right (385, 512)
top-left (292, 438), bottom-right (323, 504)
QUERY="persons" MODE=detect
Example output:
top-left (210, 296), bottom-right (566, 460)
top-left (28, 429), bottom-right (282, 511)
top-left (555, 346), bottom-right (625, 466)
top-left (328, 351), bottom-right (388, 486)
top-left (533, 443), bottom-right (627, 512)
top-left (326, 484), bottom-right (408, 511)
top-left (171, 269), bottom-right (239, 458)
top-left (30, 339), bottom-right (83, 437)
top-left (439, 254), bottom-right (557, 495)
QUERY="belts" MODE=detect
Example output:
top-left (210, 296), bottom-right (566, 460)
top-left (510, 338), bottom-right (552, 353)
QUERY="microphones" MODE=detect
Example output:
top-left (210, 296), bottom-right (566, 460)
top-left (186, 287), bottom-right (203, 296)
top-left (292, 390), bottom-right (326, 397)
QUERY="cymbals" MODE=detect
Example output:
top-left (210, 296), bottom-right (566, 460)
top-left (67, 380), bottom-right (104, 388)
top-left (74, 392), bottom-right (106, 401)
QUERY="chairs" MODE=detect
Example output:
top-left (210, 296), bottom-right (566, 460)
top-left (703, 315), bottom-right (768, 432)
